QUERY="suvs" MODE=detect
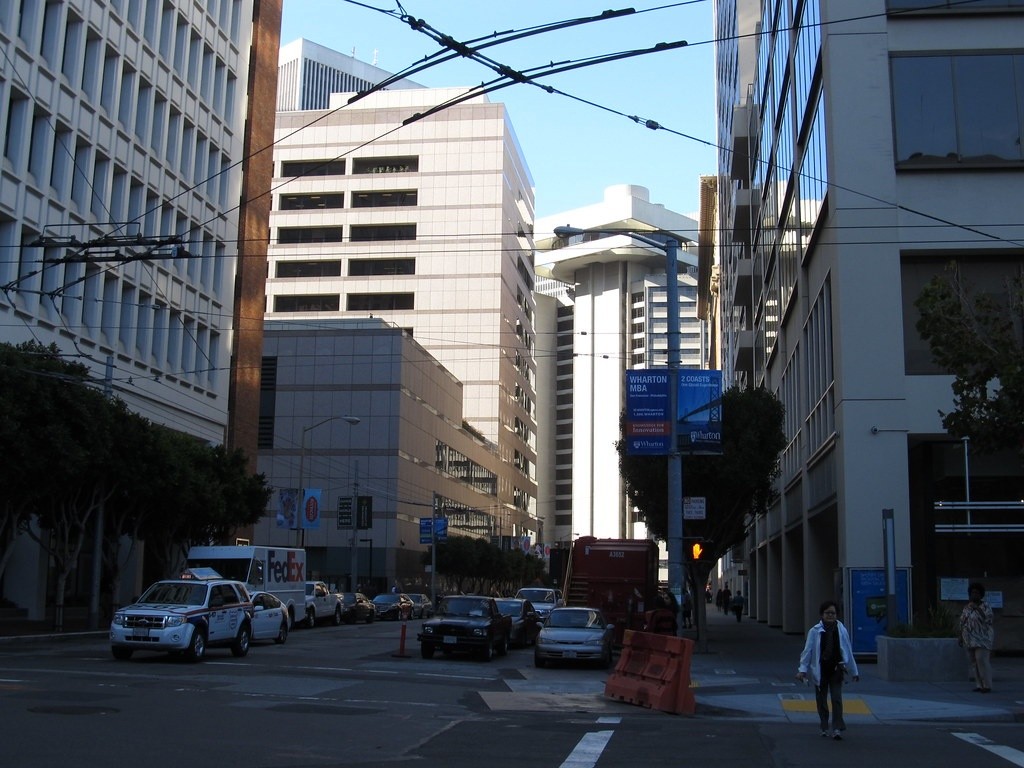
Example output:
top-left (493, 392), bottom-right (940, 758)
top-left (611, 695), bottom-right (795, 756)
top-left (110, 580), bottom-right (255, 664)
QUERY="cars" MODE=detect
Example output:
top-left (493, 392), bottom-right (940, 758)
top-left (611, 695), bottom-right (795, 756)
top-left (493, 598), bottom-right (542, 650)
top-left (341, 593), bottom-right (377, 625)
top-left (407, 594), bottom-right (434, 619)
top-left (535, 607), bottom-right (614, 668)
top-left (515, 587), bottom-right (566, 625)
top-left (372, 593), bottom-right (415, 620)
top-left (249, 591), bottom-right (292, 645)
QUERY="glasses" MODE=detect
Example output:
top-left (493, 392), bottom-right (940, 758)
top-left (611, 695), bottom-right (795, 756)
top-left (824, 612), bottom-right (836, 614)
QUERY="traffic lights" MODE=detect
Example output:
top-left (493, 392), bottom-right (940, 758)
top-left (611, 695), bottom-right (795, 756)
top-left (684, 537), bottom-right (704, 561)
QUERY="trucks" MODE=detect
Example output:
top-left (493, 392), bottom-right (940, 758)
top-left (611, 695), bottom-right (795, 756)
top-left (187, 545), bottom-right (306, 632)
top-left (566, 536), bottom-right (659, 645)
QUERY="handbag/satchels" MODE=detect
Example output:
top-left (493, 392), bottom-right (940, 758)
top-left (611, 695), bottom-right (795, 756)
top-left (831, 658), bottom-right (846, 672)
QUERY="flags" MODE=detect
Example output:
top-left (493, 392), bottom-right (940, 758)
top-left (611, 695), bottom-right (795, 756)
top-left (490, 536), bottom-right (551, 558)
top-left (275, 489), bottom-right (321, 529)
top-left (336, 495), bottom-right (372, 530)
top-left (420, 517), bottom-right (447, 544)
top-left (625, 369), bottom-right (723, 457)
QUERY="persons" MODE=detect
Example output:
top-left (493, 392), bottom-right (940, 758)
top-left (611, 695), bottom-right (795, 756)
top-left (798, 601), bottom-right (860, 740)
top-left (716, 585), bottom-right (731, 615)
top-left (706, 588), bottom-right (713, 603)
top-left (732, 590), bottom-right (747, 622)
top-left (644, 588), bottom-right (693, 637)
top-left (958, 583), bottom-right (994, 693)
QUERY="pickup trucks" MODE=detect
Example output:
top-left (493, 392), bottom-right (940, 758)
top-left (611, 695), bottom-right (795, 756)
top-left (417, 594), bottom-right (513, 662)
top-left (304, 580), bottom-right (344, 629)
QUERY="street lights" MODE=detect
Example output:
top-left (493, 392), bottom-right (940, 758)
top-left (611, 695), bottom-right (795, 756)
top-left (296, 415), bottom-right (362, 548)
top-left (520, 516), bottom-right (546, 550)
top-left (559, 533), bottom-right (579, 589)
top-left (554, 226), bottom-right (684, 637)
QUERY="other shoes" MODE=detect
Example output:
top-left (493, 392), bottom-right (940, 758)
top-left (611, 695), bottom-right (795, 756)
top-left (833, 729), bottom-right (842, 740)
top-left (822, 732), bottom-right (829, 737)
